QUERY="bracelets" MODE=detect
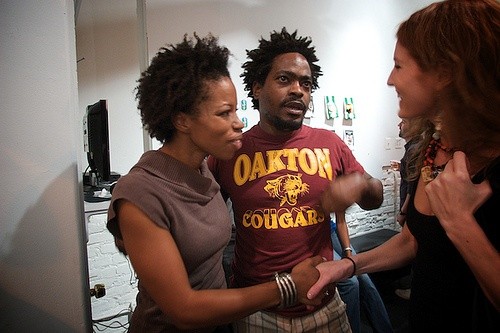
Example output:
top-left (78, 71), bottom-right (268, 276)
top-left (284, 272), bottom-right (297, 303)
top-left (281, 274), bottom-right (294, 305)
top-left (341, 257), bottom-right (356, 278)
top-left (279, 277), bottom-right (289, 306)
top-left (342, 248), bottom-right (353, 253)
top-left (274, 271), bottom-right (285, 309)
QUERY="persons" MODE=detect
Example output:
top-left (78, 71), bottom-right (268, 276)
top-left (200, 27), bottom-right (427, 333)
top-left (306, 0), bottom-right (500, 333)
top-left (105, 31), bottom-right (333, 333)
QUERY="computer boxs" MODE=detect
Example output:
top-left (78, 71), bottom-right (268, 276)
top-left (82, 172), bottom-right (121, 185)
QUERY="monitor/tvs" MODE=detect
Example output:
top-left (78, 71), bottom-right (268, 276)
top-left (87, 99), bottom-right (111, 182)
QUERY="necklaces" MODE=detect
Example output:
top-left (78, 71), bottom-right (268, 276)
top-left (421, 135), bottom-right (453, 182)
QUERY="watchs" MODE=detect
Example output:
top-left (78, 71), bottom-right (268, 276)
top-left (399, 208), bottom-right (406, 215)
